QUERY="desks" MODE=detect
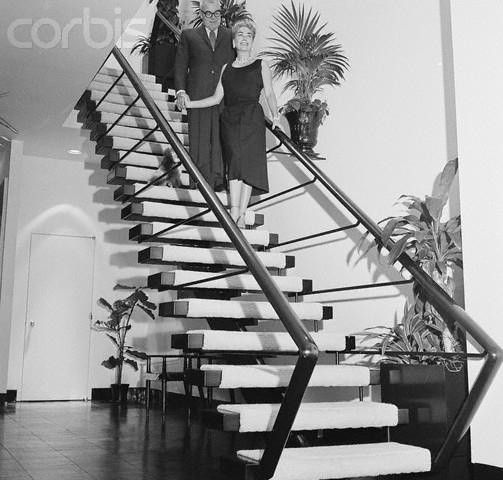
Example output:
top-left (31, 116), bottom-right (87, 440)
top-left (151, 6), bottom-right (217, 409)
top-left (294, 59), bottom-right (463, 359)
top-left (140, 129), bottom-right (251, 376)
top-left (144, 354), bottom-right (213, 427)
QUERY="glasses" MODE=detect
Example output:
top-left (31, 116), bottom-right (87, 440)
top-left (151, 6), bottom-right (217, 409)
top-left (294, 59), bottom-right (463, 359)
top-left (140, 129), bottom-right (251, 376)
top-left (201, 9), bottom-right (221, 18)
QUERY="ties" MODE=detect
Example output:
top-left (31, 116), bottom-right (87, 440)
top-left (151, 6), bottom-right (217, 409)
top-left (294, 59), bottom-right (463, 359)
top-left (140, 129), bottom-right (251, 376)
top-left (210, 30), bottom-right (216, 51)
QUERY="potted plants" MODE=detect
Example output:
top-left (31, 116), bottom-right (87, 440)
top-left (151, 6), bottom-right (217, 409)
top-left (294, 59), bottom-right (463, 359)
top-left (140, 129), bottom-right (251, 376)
top-left (92, 289), bottom-right (156, 402)
top-left (349, 158), bottom-right (467, 455)
top-left (256, 0), bottom-right (351, 156)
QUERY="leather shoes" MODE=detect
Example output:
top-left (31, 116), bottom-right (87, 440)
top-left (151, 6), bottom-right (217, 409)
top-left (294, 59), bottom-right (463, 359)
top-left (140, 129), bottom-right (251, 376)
top-left (215, 184), bottom-right (229, 193)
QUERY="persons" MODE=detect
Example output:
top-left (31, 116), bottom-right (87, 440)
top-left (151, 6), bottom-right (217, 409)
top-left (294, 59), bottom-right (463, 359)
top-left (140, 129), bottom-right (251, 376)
top-left (173, 0), bottom-right (237, 193)
top-left (179, 20), bottom-right (286, 229)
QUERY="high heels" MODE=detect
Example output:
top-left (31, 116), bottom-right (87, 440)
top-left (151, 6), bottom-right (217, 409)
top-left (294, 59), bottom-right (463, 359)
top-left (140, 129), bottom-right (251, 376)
top-left (237, 210), bottom-right (246, 229)
top-left (230, 205), bottom-right (238, 224)
